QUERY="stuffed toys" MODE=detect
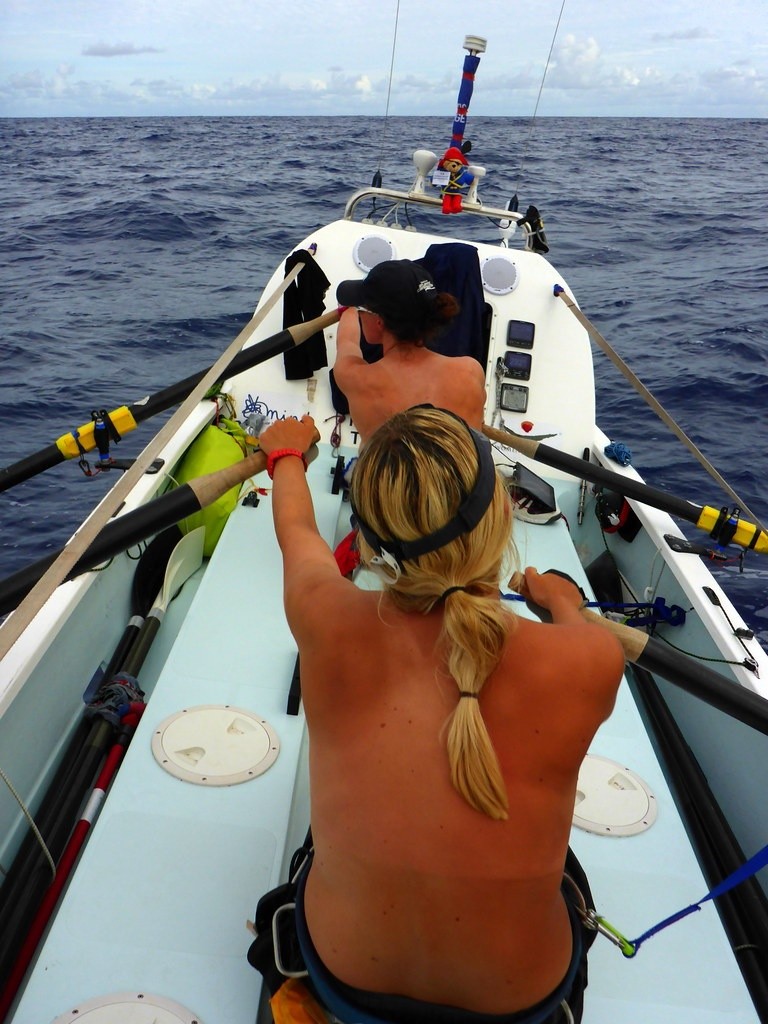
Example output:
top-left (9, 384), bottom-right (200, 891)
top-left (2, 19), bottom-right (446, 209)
top-left (429, 147), bottom-right (476, 214)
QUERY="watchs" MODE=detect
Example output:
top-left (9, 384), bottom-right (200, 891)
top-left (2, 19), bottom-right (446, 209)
top-left (265, 444), bottom-right (309, 481)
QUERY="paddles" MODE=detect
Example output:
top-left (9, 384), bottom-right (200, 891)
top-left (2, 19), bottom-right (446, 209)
top-left (482, 423), bottom-right (768, 554)
top-left (508, 572), bottom-right (768, 737)
top-left (0, 428), bottom-right (321, 617)
top-left (0, 524), bottom-right (208, 1023)
top-left (0, 310), bottom-right (341, 491)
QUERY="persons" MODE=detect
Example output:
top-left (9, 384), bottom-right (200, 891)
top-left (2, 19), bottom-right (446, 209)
top-left (332, 256), bottom-right (489, 458)
top-left (256, 402), bottom-right (627, 1023)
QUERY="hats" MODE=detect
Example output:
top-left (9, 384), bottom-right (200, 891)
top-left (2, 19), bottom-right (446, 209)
top-left (336, 258), bottom-right (443, 331)
